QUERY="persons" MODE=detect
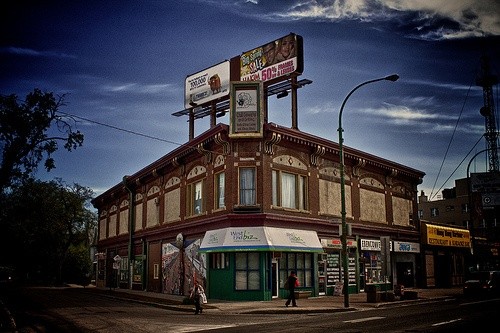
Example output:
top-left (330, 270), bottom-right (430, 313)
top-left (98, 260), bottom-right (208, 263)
top-left (285, 272), bottom-right (298, 307)
top-left (190, 280), bottom-right (204, 315)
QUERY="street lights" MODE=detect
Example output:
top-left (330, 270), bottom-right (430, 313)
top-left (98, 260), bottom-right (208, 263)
top-left (337, 73), bottom-right (400, 308)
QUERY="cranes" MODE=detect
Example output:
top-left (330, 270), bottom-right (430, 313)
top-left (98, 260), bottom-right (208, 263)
top-left (482, 84), bottom-right (500, 173)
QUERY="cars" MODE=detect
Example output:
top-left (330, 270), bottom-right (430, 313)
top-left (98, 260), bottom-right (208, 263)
top-left (461, 269), bottom-right (500, 298)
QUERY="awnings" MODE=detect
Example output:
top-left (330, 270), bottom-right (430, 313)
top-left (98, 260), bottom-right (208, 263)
top-left (199, 227), bottom-right (325, 255)
